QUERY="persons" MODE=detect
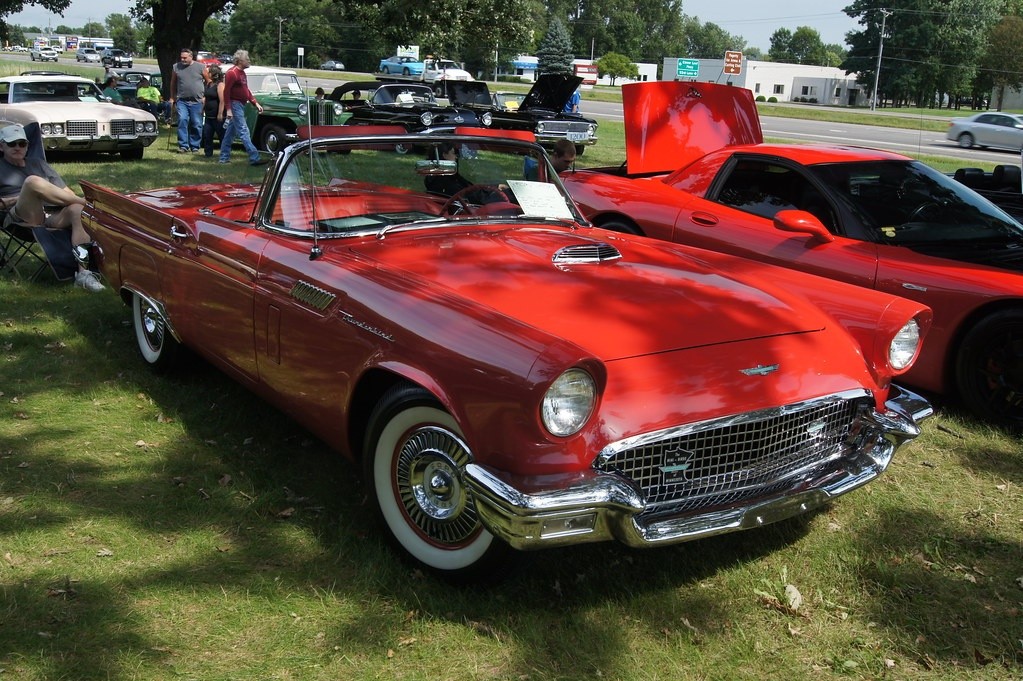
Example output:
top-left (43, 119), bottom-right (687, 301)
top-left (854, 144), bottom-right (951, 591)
top-left (527, 139), bottom-right (576, 183)
top-left (0, 126), bottom-right (105, 292)
top-left (351, 90), bottom-right (360, 99)
top-left (218, 50), bottom-right (269, 168)
top-left (199, 64), bottom-right (225, 156)
top-left (424, 143), bottom-right (519, 206)
top-left (90, 77), bottom-right (104, 93)
top-left (137, 78), bottom-right (170, 124)
top-left (105, 65), bottom-right (119, 79)
top-left (565, 90), bottom-right (580, 113)
top-left (103, 77), bottom-right (145, 109)
top-left (169, 49), bottom-right (211, 154)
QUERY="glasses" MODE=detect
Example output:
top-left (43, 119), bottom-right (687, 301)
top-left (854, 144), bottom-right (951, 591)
top-left (3, 141), bottom-right (27, 147)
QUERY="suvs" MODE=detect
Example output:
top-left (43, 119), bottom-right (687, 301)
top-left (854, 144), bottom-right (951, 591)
top-left (216, 64), bottom-right (355, 158)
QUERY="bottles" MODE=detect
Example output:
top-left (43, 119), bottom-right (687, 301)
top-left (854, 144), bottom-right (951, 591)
top-left (223, 118), bottom-right (230, 128)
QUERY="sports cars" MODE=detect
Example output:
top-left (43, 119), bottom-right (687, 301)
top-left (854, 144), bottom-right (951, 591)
top-left (548, 80), bottom-right (1023, 427)
top-left (70, 123), bottom-right (936, 587)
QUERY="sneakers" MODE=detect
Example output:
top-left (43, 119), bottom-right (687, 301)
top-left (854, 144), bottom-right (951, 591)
top-left (74, 270), bottom-right (105, 292)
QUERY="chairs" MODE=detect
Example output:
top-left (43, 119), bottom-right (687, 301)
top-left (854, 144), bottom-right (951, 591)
top-left (738, 166), bottom-right (923, 233)
top-left (95, 84), bottom-right (168, 127)
top-left (954, 164), bottom-right (1021, 193)
top-left (0, 123), bottom-right (78, 283)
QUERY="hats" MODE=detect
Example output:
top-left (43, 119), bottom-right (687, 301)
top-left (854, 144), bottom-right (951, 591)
top-left (0, 125), bottom-right (28, 143)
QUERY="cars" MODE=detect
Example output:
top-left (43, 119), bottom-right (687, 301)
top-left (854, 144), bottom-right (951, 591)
top-left (0, 69), bottom-right (161, 162)
top-left (86, 67), bottom-right (164, 104)
top-left (2, 45), bottom-right (64, 62)
top-left (320, 60), bottom-right (345, 71)
top-left (99, 48), bottom-right (133, 69)
top-left (75, 47), bottom-right (101, 63)
top-left (314, 58), bottom-right (599, 156)
top-left (194, 50), bottom-right (235, 68)
top-left (378, 55), bottom-right (433, 77)
top-left (943, 110), bottom-right (1023, 154)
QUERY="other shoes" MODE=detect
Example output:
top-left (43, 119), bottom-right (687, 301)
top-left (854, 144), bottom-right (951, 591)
top-left (250, 159), bottom-right (268, 166)
top-left (177, 148), bottom-right (184, 152)
